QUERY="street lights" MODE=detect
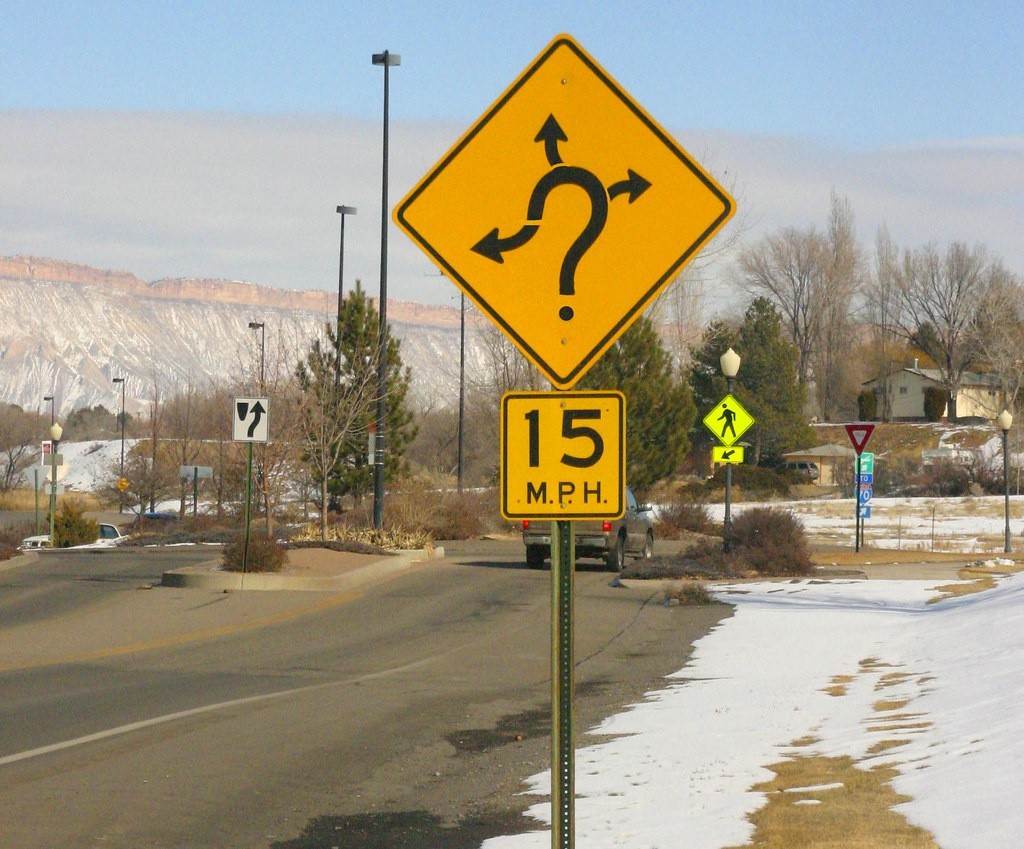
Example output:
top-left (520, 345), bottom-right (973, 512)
top-left (249, 322), bottom-right (264, 510)
top-left (720, 348), bottom-right (742, 555)
top-left (113, 379), bottom-right (124, 513)
top-left (331, 205), bottom-right (357, 512)
top-left (372, 49), bottom-right (401, 549)
top-left (997, 410), bottom-right (1014, 553)
top-left (44, 396), bottom-right (56, 507)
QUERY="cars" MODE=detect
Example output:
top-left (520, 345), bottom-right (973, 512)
top-left (143, 512), bottom-right (178, 521)
top-left (775, 460), bottom-right (819, 481)
top-left (16, 524), bottom-right (121, 554)
top-left (522, 484), bottom-right (654, 574)
